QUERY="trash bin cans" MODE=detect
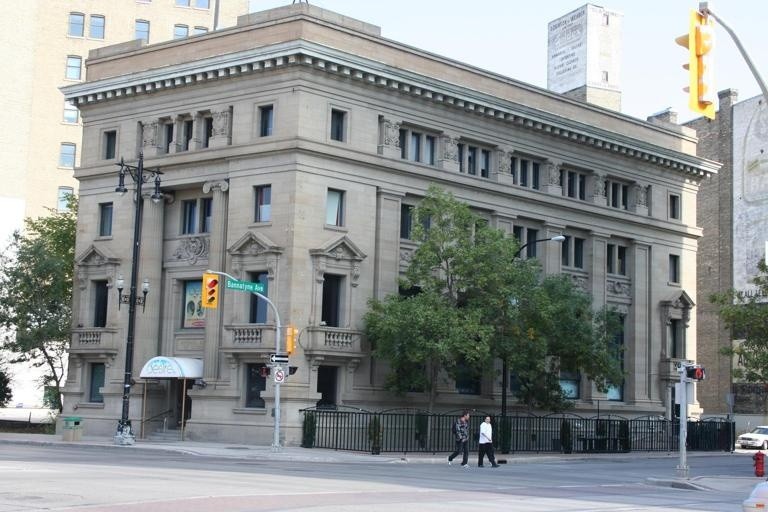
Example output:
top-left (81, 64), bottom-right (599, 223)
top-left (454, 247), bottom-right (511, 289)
top-left (62, 418), bottom-right (83, 441)
top-left (551, 439), bottom-right (561, 452)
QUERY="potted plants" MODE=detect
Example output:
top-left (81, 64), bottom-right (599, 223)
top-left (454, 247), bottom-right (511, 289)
top-left (368, 417), bottom-right (381, 454)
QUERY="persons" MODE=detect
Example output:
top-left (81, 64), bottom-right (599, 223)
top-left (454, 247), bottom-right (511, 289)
top-left (447, 411), bottom-right (472, 467)
top-left (477, 415), bottom-right (500, 467)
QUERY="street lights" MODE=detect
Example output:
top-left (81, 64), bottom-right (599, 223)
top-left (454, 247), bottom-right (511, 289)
top-left (115, 148), bottom-right (164, 441)
top-left (502, 235), bottom-right (566, 418)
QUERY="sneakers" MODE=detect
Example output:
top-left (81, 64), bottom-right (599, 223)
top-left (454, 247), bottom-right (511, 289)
top-left (446, 455), bottom-right (452, 466)
top-left (460, 463), bottom-right (470, 468)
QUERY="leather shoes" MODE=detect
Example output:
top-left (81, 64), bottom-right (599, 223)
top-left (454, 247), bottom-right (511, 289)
top-left (491, 463), bottom-right (500, 468)
top-left (477, 463), bottom-right (485, 467)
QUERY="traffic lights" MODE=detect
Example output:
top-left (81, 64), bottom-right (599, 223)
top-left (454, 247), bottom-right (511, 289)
top-left (674, 9), bottom-right (715, 120)
top-left (286, 327), bottom-right (298, 356)
top-left (201, 273), bottom-right (219, 308)
top-left (687, 366), bottom-right (705, 381)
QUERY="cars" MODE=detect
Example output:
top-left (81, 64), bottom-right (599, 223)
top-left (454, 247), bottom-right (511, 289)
top-left (736, 426), bottom-right (768, 450)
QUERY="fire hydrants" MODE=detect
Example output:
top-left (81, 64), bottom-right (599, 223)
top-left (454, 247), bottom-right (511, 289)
top-left (752, 451), bottom-right (766, 476)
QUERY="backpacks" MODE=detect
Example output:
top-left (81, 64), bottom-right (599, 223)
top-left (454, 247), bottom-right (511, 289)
top-left (452, 419), bottom-right (464, 434)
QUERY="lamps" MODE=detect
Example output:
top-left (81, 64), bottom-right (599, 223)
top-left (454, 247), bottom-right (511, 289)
top-left (194, 379), bottom-right (207, 387)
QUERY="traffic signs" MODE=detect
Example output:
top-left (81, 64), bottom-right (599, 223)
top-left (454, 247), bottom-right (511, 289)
top-left (227, 279), bottom-right (265, 293)
